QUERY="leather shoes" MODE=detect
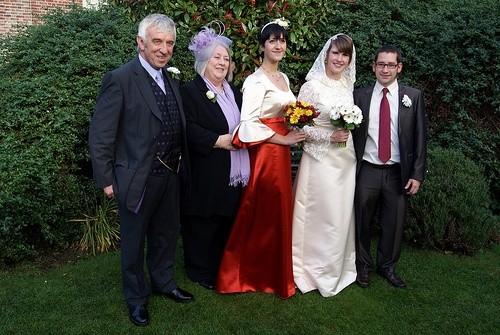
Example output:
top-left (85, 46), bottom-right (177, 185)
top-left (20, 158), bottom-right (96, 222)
top-left (376, 270), bottom-right (406, 288)
top-left (153, 287), bottom-right (195, 303)
top-left (189, 276), bottom-right (215, 290)
top-left (356, 274), bottom-right (370, 288)
top-left (128, 303), bottom-right (150, 327)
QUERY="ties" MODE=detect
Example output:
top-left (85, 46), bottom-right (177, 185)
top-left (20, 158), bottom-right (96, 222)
top-left (378, 88), bottom-right (391, 164)
top-left (156, 72), bottom-right (166, 96)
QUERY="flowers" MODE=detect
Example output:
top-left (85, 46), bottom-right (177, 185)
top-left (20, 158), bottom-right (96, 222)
top-left (167, 67), bottom-right (182, 81)
top-left (206, 90), bottom-right (218, 103)
top-left (282, 101), bottom-right (322, 149)
top-left (402, 95), bottom-right (412, 108)
top-left (328, 104), bottom-right (363, 148)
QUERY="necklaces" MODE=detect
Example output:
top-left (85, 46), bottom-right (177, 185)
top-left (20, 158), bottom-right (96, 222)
top-left (261, 65), bottom-right (282, 81)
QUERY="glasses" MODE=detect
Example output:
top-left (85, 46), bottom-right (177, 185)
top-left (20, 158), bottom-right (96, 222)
top-left (375, 62), bottom-right (398, 69)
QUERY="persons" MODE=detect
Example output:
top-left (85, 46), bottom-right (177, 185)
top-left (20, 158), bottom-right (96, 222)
top-left (291, 33), bottom-right (357, 298)
top-left (353, 45), bottom-right (428, 288)
top-left (89, 13), bottom-right (195, 327)
top-left (217, 19), bottom-right (307, 300)
top-left (179, 27), bottom-right (250, 290)
